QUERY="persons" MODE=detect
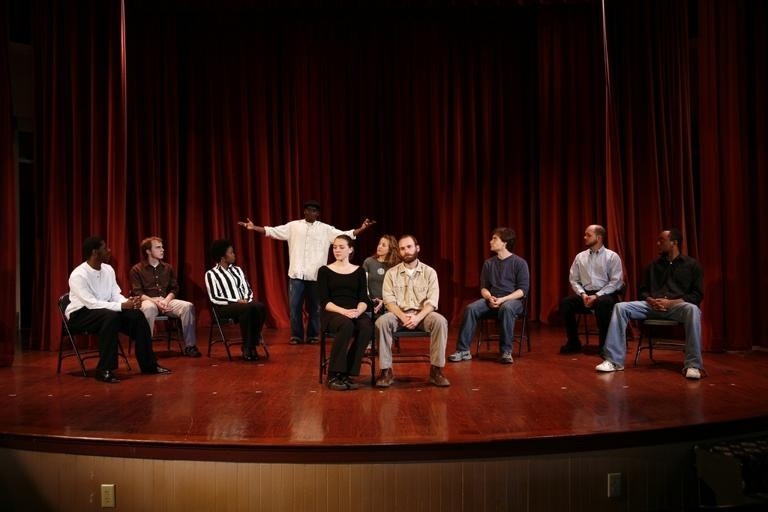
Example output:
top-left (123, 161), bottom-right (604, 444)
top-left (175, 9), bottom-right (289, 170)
top-left (204, 239), bottom-right (266, 361)
top-left (130, 237), bottom-right (202, 357)
top-left (61, 237), bottom-right (172, 383)
top-left (317, 235), bottom-right (374, 390)
top-left (559, 225), bottom-right (625, 354)
top-left (238, 203), bottom-right (377, 345)
top-left (361, 234), bottom-right (402, 363)
top-left (449, 227), bottom-right (530, 364)
top-left (375, 235), bottom-right (450, 387)
top-left (595, 228), bottom-right (704, 379)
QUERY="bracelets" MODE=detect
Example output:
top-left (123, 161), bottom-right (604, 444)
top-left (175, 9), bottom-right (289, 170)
top-left (254, 225), bottom-right (256, 231)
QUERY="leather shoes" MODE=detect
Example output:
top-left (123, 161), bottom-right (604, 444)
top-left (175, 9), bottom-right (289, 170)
top-left (95, 370), bottom-right (120, 383)
top-left (249, 349), bottom-right (260, 361)
top-left (142, 365), bottom-right (170, 374)
top-left (241, 347), bottom-right (252, 361)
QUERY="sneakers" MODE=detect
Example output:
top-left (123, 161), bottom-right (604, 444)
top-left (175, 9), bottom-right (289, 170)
top-left (346, 376), bottom-right (360, 388)
top-left (594, 360), bottom-right (624, 371)
top-left (448, 350), bottom-right (472, 361)
top-left (429, 365), bottom-right (450, 386)
top-left (685, 368), bottom-right (701, 378)
top-left (288, 337), bottom-right (302, 344)
top-left (306, 336), bottom-right (320, 344)
top-left (186, 347), bottom-right (201, 356)
top-left (560, 339), bottom-right (581, 353)
top-left (326, 375), bottom-right (346, 390)
top-left (501, 352), bottom-right (514, 362)
top-left (376, 368), bottom-right (394, 386)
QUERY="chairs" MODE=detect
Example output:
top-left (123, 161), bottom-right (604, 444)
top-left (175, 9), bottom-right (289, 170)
top-left (128, 291), bottom-right (188, 357)
top-left (575, 281), bottom-right (629, 349)
top-left (475, 288), bottom-right (531, 356)
top-left (206, 293), bottom-right (269, 361)
top-left (634, 264), bottom-right (704, 369)
top-left (317, 299), bottom-right (376, 386)
top-left (57, 293), bottom-right (132, 376)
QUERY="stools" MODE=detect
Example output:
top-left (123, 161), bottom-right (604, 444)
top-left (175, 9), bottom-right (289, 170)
top-left (391, 323), bottom-right (431, 362)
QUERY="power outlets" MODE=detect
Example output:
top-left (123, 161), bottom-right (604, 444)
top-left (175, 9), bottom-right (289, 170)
top-left (100, 483), bottom-right (116, 507)
top-left (608, 474), bottom-right (623, 498)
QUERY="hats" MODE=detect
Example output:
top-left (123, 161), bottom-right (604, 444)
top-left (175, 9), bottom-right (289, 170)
top-left (305, 201), bottom-right (321, 207)
